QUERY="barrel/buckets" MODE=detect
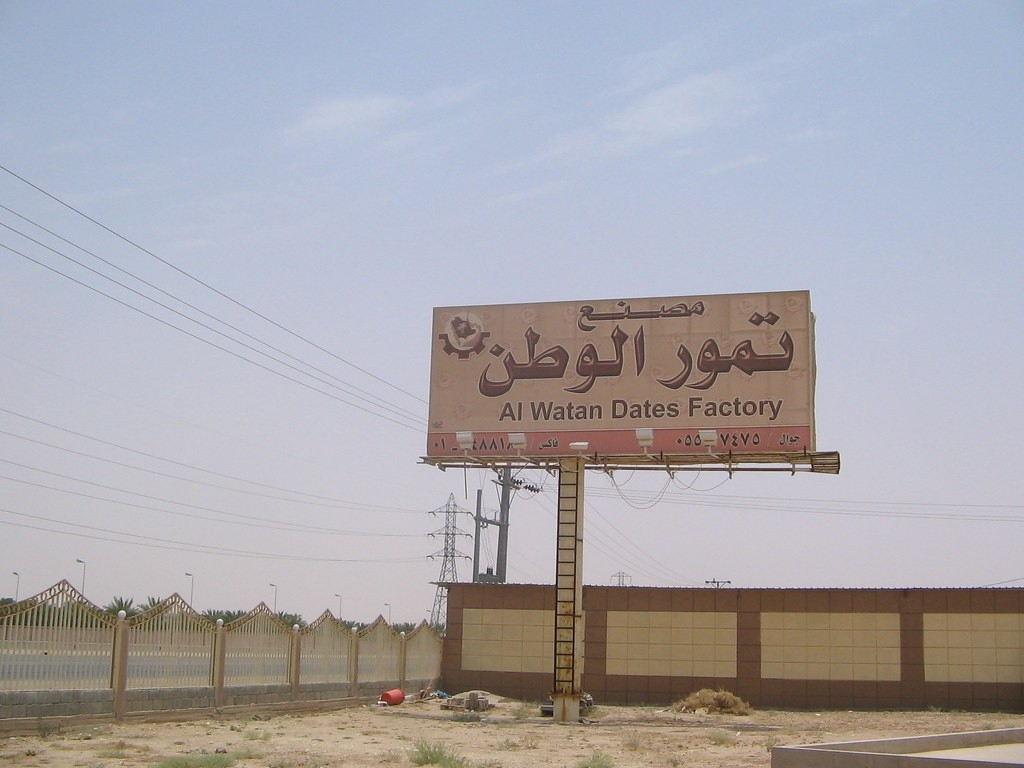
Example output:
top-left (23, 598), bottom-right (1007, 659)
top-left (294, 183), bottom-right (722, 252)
top-left (382, 689), bottom-right (404, 705)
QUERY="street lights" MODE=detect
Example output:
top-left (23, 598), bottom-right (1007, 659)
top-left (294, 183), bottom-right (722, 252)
top-left (13, 571), bottom-right (20, 603)
top-left (385, 602), bottom-right (391, 626)
top-left (335, 593), bottom-right (341, 623)
top-left (270, 583), bottom-right (277, 616)
top-left (185, 572), bottom-right (194, 607)
top-left (76, 557), bottom-right (86, 597)
